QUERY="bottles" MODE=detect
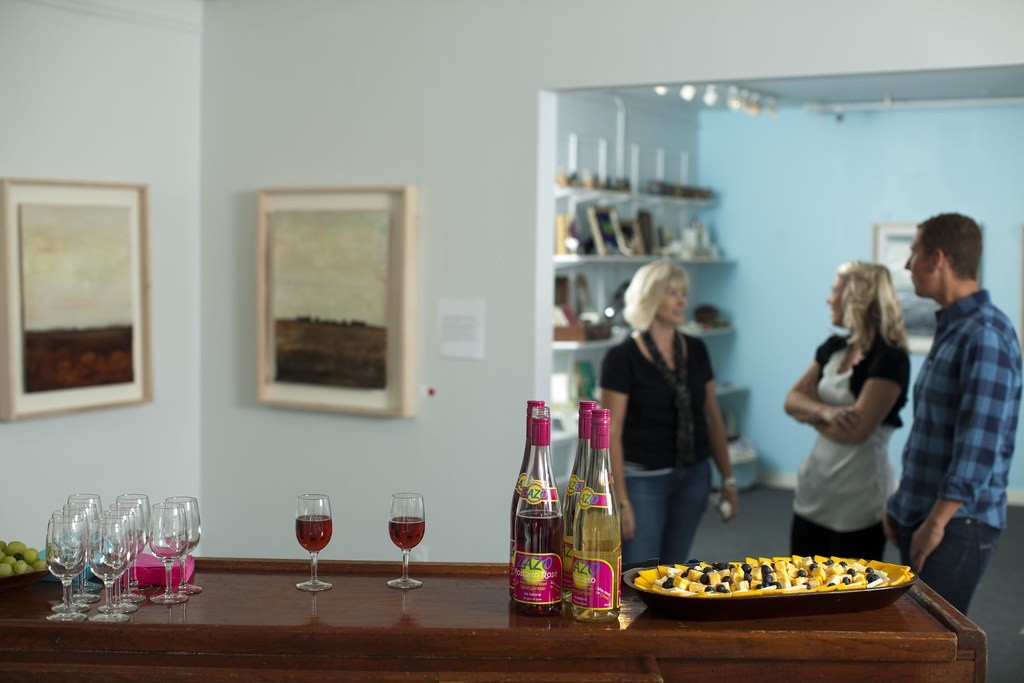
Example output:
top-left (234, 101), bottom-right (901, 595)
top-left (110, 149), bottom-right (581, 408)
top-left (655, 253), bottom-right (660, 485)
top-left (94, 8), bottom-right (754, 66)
top-left (563, 401), bottom-right (621, 621)
top-left (509, 401), bottom-right (562, 615)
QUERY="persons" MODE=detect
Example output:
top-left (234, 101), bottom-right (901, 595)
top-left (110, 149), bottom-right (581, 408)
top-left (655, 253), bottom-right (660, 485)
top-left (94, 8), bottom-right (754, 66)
top-left (600, 261), bottom-right (738, 573)
top-left (882, 214), bottom-right (1024, 616)
top-left (783, 262), bottom-right (911, 560)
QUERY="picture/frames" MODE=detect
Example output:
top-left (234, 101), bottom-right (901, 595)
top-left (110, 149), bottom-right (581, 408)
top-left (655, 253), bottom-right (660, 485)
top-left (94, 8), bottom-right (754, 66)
top-left (874, 223), bottom-right (983, 355)
top-left (255, 185), bottom-right (418, 416)
top-left (587, 205), bottom-right (627, 256)
top-left (0, 178), bottom-right (153, 424)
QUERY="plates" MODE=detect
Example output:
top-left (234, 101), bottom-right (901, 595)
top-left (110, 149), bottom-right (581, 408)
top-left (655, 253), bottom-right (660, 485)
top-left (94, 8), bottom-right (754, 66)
top-left (0, 567), bottom-right (51, 589)
top-left (623, 562), bottom-right (918, 609)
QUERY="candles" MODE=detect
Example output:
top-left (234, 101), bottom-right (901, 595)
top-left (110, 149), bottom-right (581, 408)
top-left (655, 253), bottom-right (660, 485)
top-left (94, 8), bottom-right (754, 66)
top-left (569, 100), bottom-right (689, 193)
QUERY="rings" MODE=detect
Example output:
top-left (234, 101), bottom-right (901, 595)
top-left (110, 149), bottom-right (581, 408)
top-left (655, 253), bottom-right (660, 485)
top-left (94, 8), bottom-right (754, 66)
top-left (842, 412), bottom-right (849, 418)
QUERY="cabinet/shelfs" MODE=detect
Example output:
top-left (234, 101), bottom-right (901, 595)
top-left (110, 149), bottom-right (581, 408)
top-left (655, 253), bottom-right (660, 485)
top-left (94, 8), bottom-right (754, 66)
top-left (549, 189), bottom-right (748, 442)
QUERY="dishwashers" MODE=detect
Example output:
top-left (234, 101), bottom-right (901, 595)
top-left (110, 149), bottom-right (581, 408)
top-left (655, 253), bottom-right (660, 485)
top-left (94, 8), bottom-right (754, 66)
top-left (720, 501), bottom-right (732, 517)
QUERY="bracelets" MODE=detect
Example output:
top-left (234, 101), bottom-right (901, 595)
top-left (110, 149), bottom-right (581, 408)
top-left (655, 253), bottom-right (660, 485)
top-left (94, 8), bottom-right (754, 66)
top-left (724, 479), bottom-right (736, 486)
top-left (620, 501), bottom-right (628, 507)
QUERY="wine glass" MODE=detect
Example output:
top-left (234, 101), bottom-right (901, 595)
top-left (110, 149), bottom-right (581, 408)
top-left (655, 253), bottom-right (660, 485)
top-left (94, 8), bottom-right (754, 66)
top-left (296, 495), bottom-right (333, 591)
top-left (148, 497), bottom-right (202, 604)
top-left (88, 494), bottom-right (151, 622)
top-left (46, 494), bottom-right (102, 622)
top-left (387, 494), bottom-right (424, 589)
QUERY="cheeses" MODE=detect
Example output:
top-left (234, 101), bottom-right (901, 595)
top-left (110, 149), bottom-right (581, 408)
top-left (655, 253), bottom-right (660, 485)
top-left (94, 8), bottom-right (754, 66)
top-left (633, 555), bottom-right (914, 597)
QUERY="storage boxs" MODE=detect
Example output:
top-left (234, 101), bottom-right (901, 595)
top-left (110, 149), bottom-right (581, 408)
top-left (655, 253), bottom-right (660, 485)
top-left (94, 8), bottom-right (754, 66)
top-left (707, 444), bottom-right (757, 489)
top-left (555, 276), bottom-right (610, 342)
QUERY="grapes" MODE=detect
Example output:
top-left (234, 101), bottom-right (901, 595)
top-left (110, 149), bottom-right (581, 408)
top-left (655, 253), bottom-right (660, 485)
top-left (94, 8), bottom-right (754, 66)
top-left (0, 540), bottom-right (47, 578)
